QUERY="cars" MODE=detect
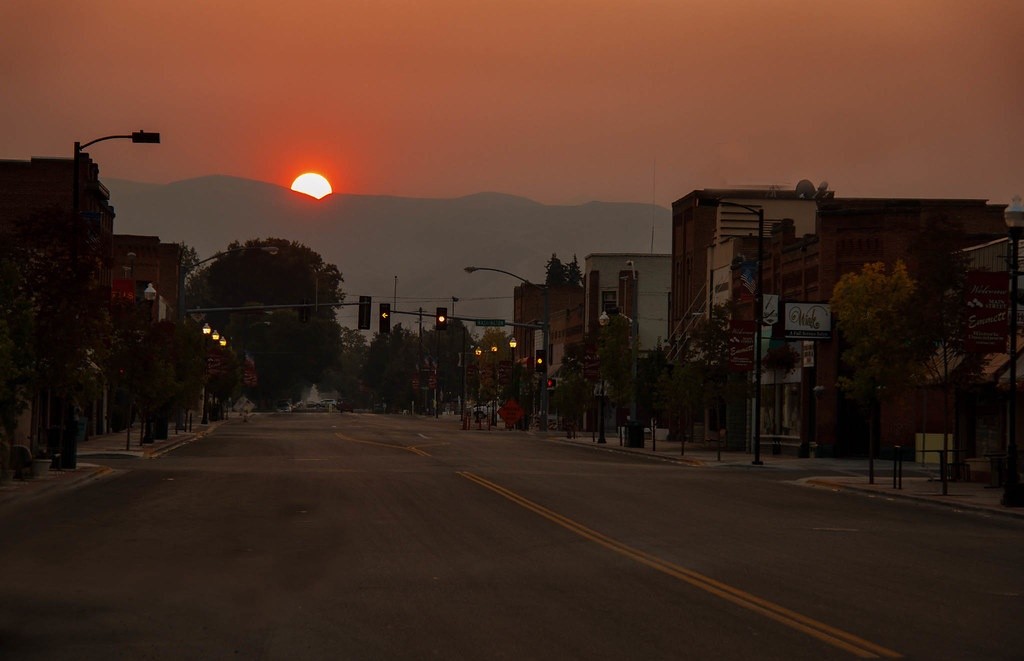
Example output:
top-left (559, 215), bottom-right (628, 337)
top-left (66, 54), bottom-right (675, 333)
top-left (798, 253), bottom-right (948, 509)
top-left (277, 399), bottom-right (354, 413)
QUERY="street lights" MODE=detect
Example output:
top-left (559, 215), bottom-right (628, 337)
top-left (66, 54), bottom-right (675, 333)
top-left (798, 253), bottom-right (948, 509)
top-left (598, 311), bottom-right (609, 443)
top-left (142, 283), bottom-right (156, 443)
top-left (697, 198), bottom-right (763, 465)
top-left (48, 130), bottom-right (159, 470)
top-left (464, 267), bottom-right (548, 432)
top-left (475, 337), bottom-right (517, 431)
top-left (175, 247), bottom-right (279, 430)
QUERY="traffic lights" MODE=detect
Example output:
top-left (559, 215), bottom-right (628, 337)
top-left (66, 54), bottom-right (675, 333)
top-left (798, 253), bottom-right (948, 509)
top-left (436, 307), bottom-right (447, 330)
top-left (379, 303), bottom-right (390, 335)
top-left (536, 350), bottom-right (546, 372)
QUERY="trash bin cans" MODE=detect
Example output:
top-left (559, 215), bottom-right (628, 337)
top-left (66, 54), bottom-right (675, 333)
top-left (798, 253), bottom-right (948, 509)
top-left (209, 404), bottom-right (222, 421)
top-left (624, 421), bottom-right (645, 448)
top-left (809, 442), bottom-right (818, 458)
top-left (990, 453), bottom-right (1008, 487)
top-left (155, 417), bottom-right (167, 440)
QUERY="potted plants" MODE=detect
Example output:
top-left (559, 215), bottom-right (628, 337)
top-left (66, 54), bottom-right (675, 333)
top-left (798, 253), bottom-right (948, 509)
top-left (31, 447), bottom-right (52, 477)
